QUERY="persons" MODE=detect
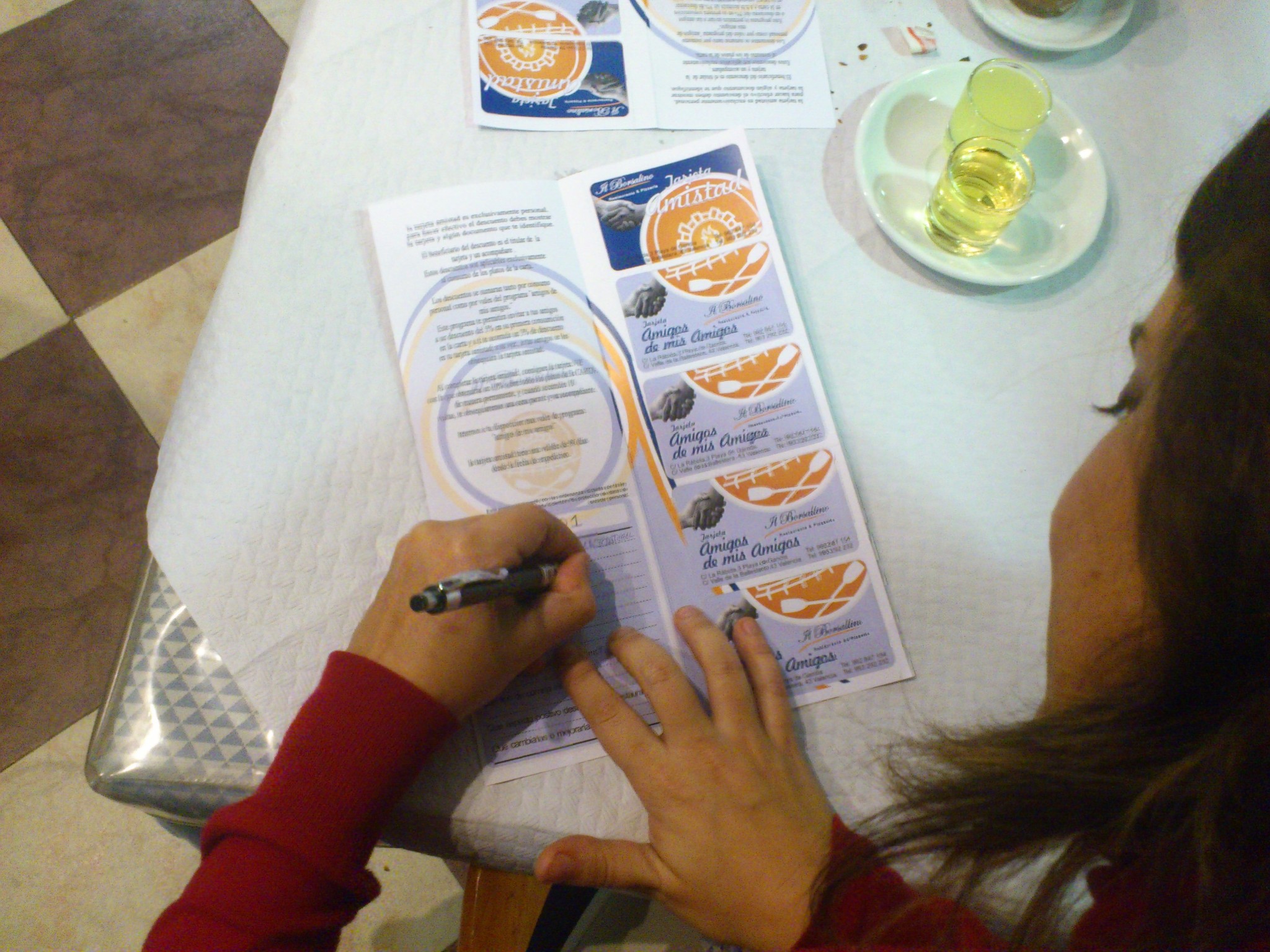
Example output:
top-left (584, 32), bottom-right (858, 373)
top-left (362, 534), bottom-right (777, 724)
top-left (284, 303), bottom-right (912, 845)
top-left (141, 111), bottom-right (1269, 952)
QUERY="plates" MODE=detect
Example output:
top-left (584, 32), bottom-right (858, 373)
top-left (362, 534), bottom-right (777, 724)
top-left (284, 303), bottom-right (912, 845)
top-left (853, 60), bottom-right (1108, 287)
top-left (970, 0), bottom-right (1134, 51)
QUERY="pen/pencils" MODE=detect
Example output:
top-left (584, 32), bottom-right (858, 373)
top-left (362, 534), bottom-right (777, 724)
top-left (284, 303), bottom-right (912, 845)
top-left (411, 563), bottom-right (558, 613)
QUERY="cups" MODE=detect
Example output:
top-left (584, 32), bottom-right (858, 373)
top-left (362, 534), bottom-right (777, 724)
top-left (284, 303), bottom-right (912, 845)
top-left (942, 58), bottom-right (1054, 152)
top-left (1012, 0), bottom-right (1078, 19)
top-left (922, 135), bottom-right (1037, 258)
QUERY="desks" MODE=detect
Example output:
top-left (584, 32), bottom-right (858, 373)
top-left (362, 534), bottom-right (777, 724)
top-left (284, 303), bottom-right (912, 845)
top-left (84, 0), bottom-right (1270, 952)
